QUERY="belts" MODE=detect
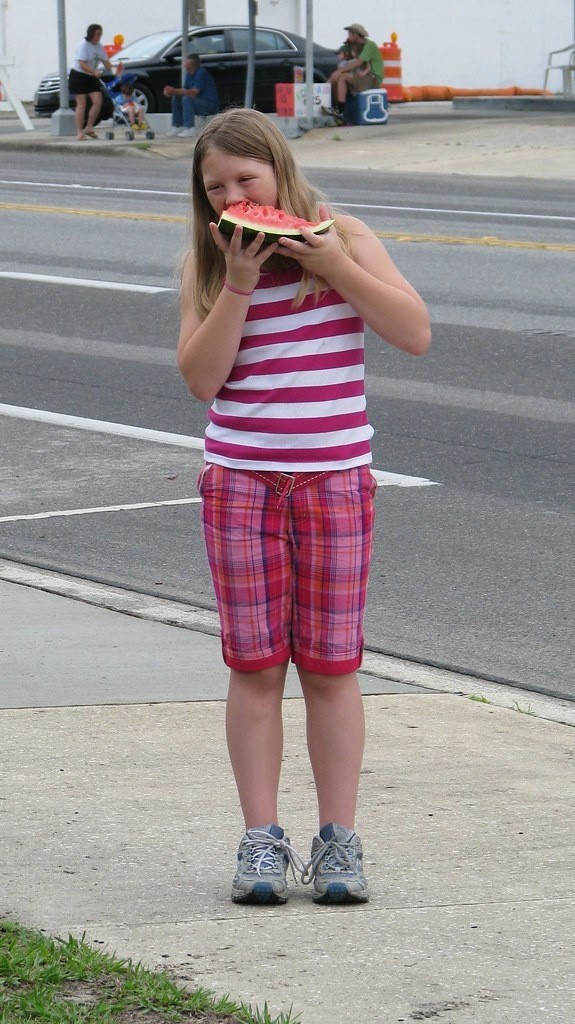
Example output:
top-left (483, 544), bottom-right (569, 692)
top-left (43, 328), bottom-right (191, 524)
top-left (242, 467), bottom-right (336, 510)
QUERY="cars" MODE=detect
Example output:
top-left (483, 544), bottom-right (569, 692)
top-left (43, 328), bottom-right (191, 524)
top-left (33, 26), bottom-right (343, 115)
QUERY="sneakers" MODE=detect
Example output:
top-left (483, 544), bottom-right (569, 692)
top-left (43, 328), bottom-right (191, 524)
top-left (300, 822), bottom-right (370, 903)
top-left (321, 105), bottom-right (343, 117)
top-left (229, 821), bottom-right (310, 902)
top-left (179, 127), bottom-right (197, 138)
top-left (167, 128), bottom-right (184, 137)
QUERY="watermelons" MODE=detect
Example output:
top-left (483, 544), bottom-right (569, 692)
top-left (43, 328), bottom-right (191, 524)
top-left (217, 199), bottom-right (336, 247)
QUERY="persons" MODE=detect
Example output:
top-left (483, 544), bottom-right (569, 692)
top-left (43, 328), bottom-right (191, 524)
top-left (114, 86), bottom-right (149, 130)
top-left (68, 23), bottom-right (111, 140)
top-left (321, 23), bottom-right (383, 115)
top-left (163, 54), bottom-right (219, 137)
top-left (176, 105), bottom-right (432, 898)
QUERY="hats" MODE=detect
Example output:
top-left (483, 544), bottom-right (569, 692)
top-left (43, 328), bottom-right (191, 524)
top-left (334, 46), bottom-right (350, 54)
top-left (344, 24), bottom-right (369, 37)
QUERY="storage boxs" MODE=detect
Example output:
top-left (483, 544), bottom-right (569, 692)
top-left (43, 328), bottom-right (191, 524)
top-left (276, 82), bottom-right (332, 118)
top-left (344, 88), bottom-right (388, 126)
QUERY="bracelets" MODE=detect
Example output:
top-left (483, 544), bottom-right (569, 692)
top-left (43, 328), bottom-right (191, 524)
top-left (225, 280), bottom-right (254, 295)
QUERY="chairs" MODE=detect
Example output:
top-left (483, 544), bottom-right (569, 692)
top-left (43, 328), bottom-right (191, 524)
top-left (543, 43), bottom-right (575, 99)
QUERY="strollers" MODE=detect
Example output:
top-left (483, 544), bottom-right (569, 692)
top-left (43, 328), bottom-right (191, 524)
top-left (99, 69), bottom-right (154, 141)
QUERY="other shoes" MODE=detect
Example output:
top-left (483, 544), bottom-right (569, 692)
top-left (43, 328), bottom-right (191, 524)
top-left (77, 133), bottom-right (88, 141)
top-left (131, 124), bottom-right (140, 130)
top-left (84, 129), bottom-right (97, 138)
top-left (138, 123), bottom-right (148, 129)
